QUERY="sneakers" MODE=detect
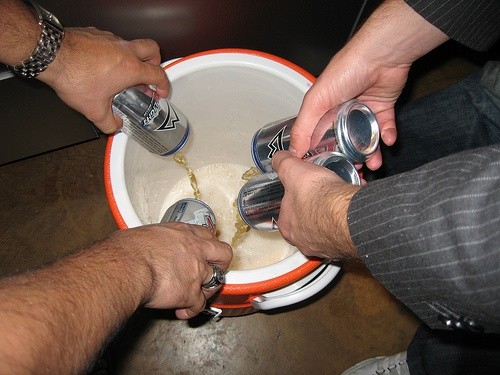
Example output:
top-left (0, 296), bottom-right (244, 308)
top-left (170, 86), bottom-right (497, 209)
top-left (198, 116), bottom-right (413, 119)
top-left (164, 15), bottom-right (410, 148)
top-left (340, 350), bottom-right (411, 375)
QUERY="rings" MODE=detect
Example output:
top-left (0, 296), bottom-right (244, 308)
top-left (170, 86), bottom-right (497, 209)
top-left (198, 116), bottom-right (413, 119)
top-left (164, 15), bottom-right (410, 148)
top-left (203, 262), bottom-right (226, 292)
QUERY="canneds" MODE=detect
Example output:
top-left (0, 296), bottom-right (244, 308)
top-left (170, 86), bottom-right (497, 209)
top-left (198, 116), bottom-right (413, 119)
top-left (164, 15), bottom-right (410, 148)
top-left (251, 98), bottom-right (380, 166)
top-left (236, 152), bottom-right (360, 231)
top-left (111, 82), bottom-right (191, 156)
top-left (160, 199), bottom-right (216, 236)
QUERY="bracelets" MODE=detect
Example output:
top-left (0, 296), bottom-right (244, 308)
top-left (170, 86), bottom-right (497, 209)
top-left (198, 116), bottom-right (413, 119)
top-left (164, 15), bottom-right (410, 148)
top-left (5, 1), bottom-right (66, 80)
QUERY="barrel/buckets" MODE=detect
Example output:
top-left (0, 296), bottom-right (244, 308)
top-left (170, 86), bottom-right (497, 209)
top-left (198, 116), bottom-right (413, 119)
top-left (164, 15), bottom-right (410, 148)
top-left (103, 48), bottom-right (370, 322)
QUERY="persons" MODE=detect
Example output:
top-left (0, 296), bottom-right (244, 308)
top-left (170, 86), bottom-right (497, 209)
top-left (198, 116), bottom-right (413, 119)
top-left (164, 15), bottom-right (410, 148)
top-left (0, 0), bottom-right (170, 135)
top-left (0, 223), bottom-right (239, 375)
top-left (269, 0), bottom-right (500, 375)
